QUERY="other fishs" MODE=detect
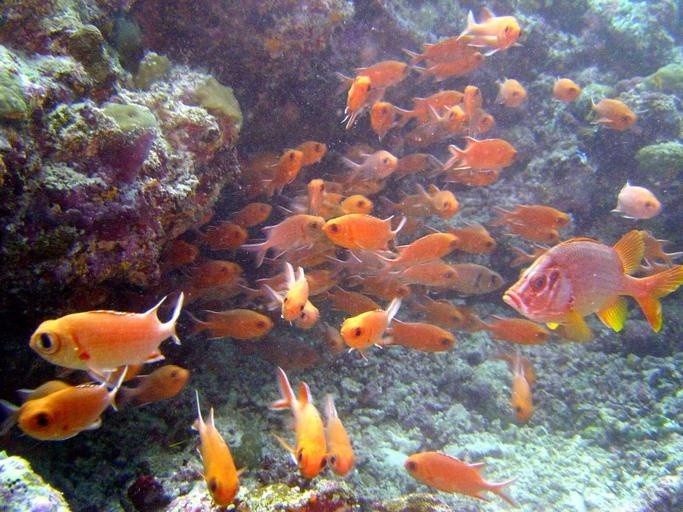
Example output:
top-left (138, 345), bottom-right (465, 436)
top-left (0, 6), bottom-right (683, 505)
